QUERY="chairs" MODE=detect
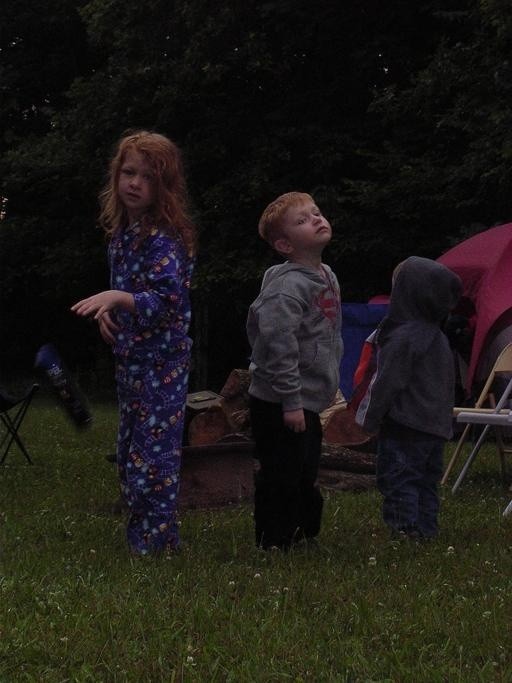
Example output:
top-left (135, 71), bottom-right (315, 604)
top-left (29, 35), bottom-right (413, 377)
top-left (439, 342), bottom-right (512, 495)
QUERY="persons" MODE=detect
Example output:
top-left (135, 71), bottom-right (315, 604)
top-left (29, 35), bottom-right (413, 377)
top-left (355, 251), bottom-right (467, 546)
top-left (245, 189), bottom-right (345, 553)
top-left (67, 125), bottom-right (200, 564)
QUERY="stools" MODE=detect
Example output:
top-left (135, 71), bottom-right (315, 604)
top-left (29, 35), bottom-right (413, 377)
top-left (0, 381), bottom-right (40, 467)
